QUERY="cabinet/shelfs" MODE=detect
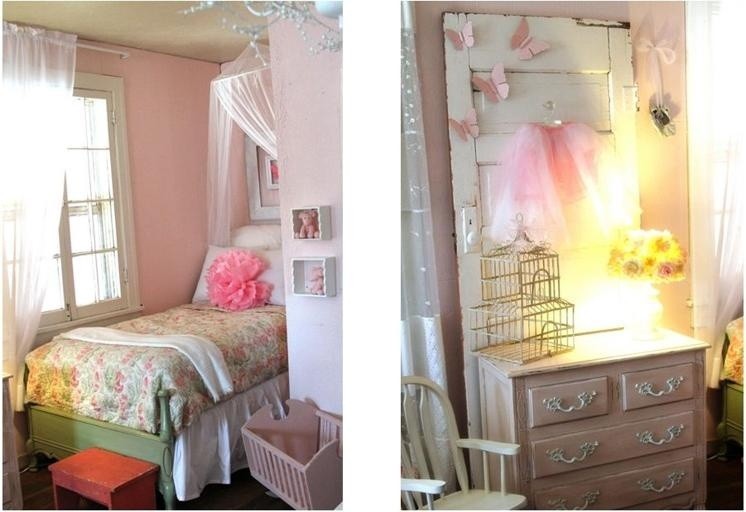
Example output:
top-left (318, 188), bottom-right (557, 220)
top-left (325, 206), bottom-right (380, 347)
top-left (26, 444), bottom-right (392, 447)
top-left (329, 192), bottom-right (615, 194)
top-left (475, 325), bottom-right (714, 509)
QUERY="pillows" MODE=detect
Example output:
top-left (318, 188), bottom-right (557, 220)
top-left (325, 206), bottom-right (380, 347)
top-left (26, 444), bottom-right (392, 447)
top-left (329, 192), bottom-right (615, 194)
top-left (190, 243), bottom-right (287, 307)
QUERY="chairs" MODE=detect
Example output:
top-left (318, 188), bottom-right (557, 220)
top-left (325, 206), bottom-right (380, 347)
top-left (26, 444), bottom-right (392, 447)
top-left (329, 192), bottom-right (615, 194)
top-left (401, 375), bottom-right (527, 510)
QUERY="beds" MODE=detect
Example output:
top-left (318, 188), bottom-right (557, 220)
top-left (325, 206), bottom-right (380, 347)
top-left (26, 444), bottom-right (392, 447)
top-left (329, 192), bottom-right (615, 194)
top-left (21, 300), bottom-right (288, 510)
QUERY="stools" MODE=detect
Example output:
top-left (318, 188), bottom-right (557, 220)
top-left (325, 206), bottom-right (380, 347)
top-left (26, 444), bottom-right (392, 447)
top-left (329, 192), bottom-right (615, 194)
top-left (47, 446), bottom-right (161, 509)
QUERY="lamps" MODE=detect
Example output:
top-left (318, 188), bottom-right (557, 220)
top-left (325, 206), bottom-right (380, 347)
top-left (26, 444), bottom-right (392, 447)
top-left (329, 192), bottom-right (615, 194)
top-left (607, 229), bottom-right (688, 343)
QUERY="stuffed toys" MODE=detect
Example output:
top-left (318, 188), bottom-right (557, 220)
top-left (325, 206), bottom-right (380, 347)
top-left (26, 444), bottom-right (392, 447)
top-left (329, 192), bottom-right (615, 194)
top-left (306, 267), bottom-right (325, 294)
top-left (298, 210), bottom-right (320, 239)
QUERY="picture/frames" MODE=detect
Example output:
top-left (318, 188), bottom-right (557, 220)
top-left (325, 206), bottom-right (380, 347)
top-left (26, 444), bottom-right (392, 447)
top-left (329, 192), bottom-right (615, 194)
top-left (264, 156), bottom-right (279, 190)
top-left (244, 132), bottom-right (281, 222)
